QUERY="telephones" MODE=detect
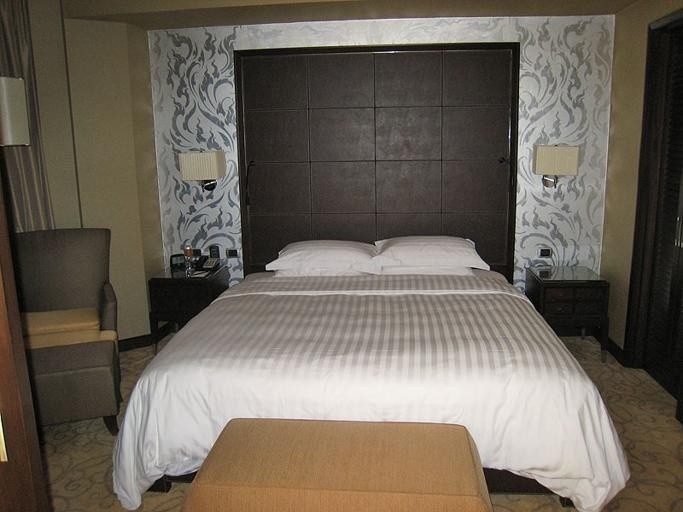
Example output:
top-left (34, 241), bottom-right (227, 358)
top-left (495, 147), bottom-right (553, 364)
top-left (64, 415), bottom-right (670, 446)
top-left (195, 256), bottom-right (220, 270)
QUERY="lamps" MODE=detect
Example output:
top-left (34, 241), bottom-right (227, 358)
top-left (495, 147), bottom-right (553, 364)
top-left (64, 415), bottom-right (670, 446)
top-left (0, 77), bottom-right (31, 150)
top-left (177, 151), bottom-right (227, 190)
top-left (531, 143), bottom-right (580, 191)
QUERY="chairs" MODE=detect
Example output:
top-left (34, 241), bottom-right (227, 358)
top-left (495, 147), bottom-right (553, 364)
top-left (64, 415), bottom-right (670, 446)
top-left (7, 224), bottom-right (123, 436)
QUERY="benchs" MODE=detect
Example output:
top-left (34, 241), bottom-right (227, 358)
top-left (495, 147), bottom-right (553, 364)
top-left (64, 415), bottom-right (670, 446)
top-left (179, 415), bottom-right (494, 512)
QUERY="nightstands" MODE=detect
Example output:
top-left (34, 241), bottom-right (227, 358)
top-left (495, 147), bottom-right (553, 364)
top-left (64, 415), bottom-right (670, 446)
top-left (525, 266), bottom-right (610, 338)
top-left (147, 261), bottom-right (232, 355)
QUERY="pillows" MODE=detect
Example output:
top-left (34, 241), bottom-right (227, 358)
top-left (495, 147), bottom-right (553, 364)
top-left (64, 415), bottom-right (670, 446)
top-left (266, 232), bottom-right (491, 276)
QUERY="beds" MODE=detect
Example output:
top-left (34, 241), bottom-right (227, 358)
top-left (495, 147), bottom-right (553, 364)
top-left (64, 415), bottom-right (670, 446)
top-left (132, 271), bottom-right (596, 510)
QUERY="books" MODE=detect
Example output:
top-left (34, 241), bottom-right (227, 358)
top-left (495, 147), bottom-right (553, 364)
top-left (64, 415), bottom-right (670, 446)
top-left (189, 269), bottom-right (211, 278)
